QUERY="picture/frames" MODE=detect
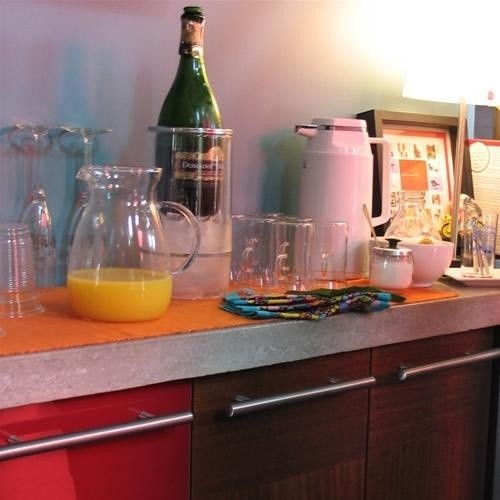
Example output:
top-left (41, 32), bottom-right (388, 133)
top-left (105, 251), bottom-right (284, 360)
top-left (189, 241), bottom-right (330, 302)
top-left (365, 111), bottom-right (475, 256)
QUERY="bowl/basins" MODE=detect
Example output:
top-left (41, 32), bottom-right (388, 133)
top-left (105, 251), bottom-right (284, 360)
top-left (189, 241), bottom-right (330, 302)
top-left (397, 241), bottom-right (455, 288)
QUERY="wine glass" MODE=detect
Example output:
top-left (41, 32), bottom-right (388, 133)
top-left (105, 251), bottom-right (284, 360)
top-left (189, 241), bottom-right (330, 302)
top-left (60, 126), bottom-right (112, 265)
top-left (14, 122), bottom-right (60, 268)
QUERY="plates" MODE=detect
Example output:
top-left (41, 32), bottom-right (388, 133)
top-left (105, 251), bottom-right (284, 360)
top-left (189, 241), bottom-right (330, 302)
top-left (442, 266), bottom-right (500, 288)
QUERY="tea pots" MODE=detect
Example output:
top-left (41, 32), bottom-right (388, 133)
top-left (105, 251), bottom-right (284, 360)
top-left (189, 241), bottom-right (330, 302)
top-left (63, 162), bottom-right (206, 325)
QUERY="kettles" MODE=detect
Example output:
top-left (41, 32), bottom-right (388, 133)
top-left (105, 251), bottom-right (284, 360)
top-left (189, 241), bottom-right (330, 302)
top-left (293, 117), bottom-right (393, 281)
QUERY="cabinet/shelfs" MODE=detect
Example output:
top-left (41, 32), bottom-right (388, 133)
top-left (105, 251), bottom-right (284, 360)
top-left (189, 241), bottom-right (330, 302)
top-left (1, 378), bottom-right (191, 499)
top-left (191, 349), bottom-right (370, 499)
top-left (369, 326), bottom-right (499, 500)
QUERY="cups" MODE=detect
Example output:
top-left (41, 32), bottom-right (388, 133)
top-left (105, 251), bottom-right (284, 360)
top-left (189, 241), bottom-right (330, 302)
top-left (462, 212), bottom-right (499, 278)
top-left (146, 126), bottom-right (234, 302)
top-left (0, 223), bottom-right (45, 318)
top-left (231, 214), bottom-right (352, 293)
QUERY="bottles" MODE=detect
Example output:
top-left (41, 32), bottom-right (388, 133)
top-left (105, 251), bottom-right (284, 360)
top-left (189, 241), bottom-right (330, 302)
top-left (369, 239), bottom-right (414, 292)
top-left (153, 5), bottom-right (226, 221)
